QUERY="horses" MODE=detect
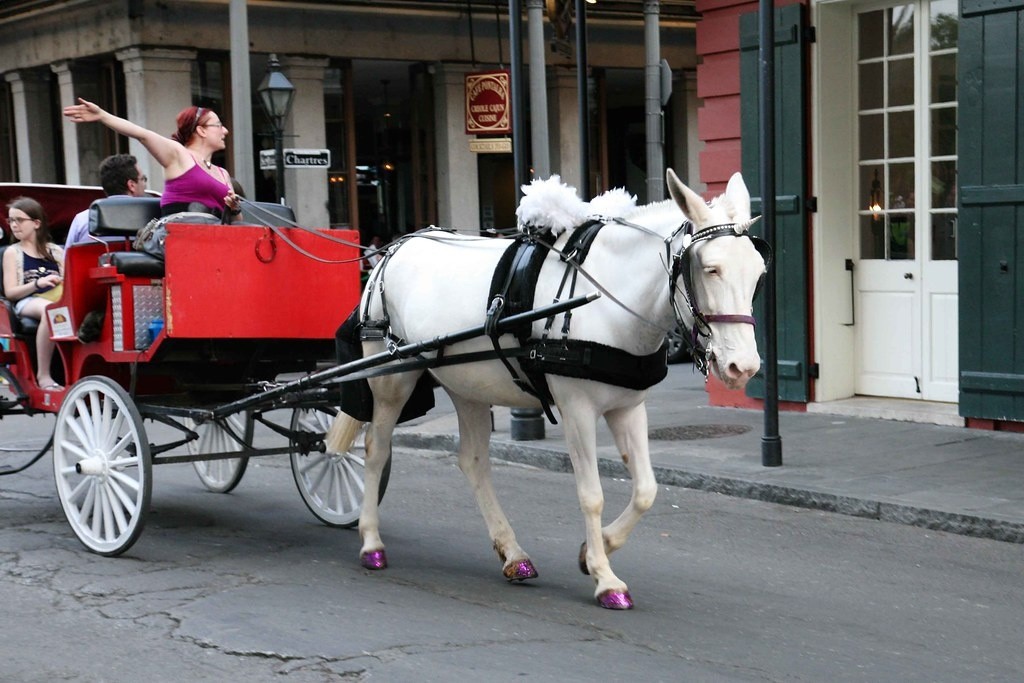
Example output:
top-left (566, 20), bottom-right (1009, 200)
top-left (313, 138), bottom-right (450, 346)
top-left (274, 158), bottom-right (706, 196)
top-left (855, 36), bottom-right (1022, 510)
top-left (324, 167), bottom-right (767, 608)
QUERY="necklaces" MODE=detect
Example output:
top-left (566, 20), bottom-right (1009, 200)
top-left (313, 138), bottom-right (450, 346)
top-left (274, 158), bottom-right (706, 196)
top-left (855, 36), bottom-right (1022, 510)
top-left (199, 156), bottom-right (213, 168)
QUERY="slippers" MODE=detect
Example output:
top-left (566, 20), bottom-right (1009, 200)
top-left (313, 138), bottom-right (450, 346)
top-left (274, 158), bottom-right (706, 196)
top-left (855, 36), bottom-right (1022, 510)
top-left (42, 383), bottom-right (65, 392)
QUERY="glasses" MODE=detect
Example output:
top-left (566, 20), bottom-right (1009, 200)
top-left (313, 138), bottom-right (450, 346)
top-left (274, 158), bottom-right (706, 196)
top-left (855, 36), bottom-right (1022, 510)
top-left (131, 175), bottom-right (148, 181)
top-left (200, 124), bottom-right (224, 128)
top-left (5, 216), bottom-right (34, 223)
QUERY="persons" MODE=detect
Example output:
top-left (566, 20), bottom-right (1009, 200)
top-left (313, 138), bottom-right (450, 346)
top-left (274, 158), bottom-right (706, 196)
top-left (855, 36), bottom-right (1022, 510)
top-left (61, 94), bottom-right (243, 222)
top-left (1, 196), bottom-right (70, 394)
top-left (61, 152), bottom-right (148, 292)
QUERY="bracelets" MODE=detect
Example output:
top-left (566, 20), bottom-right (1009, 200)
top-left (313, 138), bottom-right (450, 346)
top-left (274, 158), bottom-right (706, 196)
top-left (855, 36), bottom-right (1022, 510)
top-left (34, 278), bottom-right (44, 290)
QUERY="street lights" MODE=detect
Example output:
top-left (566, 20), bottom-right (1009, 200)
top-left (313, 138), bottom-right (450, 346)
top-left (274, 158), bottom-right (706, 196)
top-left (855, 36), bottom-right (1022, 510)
top-left (256, 52), bottom-right (296, 206)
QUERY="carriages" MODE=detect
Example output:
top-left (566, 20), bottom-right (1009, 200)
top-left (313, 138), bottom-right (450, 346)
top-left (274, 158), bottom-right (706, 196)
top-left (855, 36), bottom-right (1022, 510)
top-left (0, 168), bottom-right (772, 611)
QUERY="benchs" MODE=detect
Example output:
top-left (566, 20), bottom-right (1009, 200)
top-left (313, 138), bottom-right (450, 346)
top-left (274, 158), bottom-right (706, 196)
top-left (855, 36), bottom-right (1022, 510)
top-left (88, 197), bottom-right (298, 277)
